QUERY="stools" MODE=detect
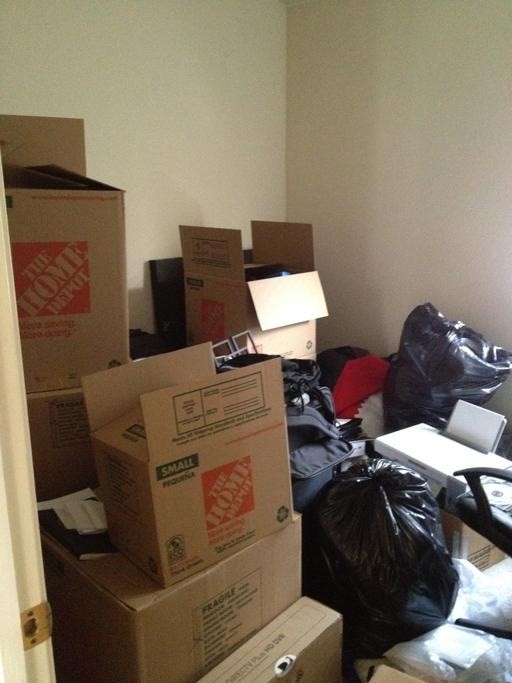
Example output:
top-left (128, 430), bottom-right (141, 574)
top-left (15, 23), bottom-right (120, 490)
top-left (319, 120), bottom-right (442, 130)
top-left (438, 461), bottom-right (511, 559)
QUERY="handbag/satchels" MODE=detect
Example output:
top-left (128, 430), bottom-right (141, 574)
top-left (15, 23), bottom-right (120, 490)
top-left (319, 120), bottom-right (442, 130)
top-left (218, 345), bottom-right (398, 512)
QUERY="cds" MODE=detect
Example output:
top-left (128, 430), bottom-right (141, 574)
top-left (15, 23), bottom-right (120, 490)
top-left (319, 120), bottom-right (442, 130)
top-left (480, 479), bottom-right (511, 511)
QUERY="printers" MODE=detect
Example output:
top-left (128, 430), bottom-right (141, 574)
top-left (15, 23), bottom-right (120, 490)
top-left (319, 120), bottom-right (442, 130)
top-left (371, 401), bottom-right (512, 506)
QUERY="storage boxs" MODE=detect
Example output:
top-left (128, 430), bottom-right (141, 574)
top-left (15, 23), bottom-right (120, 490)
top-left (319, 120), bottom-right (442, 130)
top-left (182, 594), bottom-right (345, 682)
top-left (180, 219), bottom-right (329, 364)
top-left (0, 115), bottom-right (131, 388)
top-left (81, 340), bottom-right (296, 589)
top-left (40, 510), bottom-right (302, 682)
top-left (439, 513), bottom-right (508, 572)
top-left (28, 389), bottom-right (102, 500)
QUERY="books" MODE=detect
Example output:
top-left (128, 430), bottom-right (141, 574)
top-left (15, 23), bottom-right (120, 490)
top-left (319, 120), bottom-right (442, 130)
top-left (37, 494), bottom-right (121, 560)
